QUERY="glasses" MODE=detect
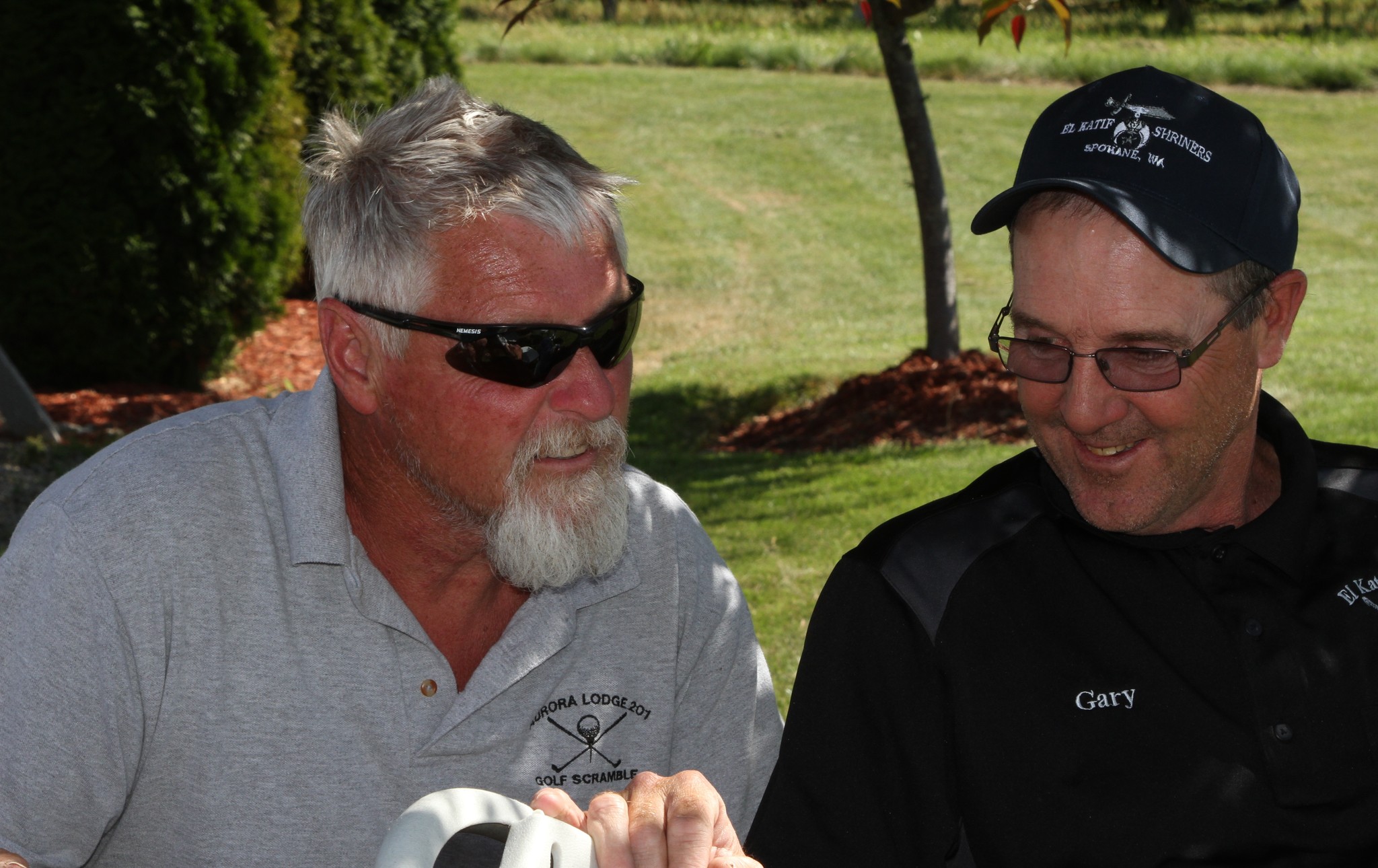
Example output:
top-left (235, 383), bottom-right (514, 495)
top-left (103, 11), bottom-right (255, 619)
top-left (989, 287), bottom-right (1252, 393)
top-left (340, 274), bottom-right (645, 388)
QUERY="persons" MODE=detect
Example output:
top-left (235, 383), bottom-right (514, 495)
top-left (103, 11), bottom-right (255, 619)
top-left (0, 90), bottom-right (785, 867)
top-left (741, 63), bottom-right (1378, 859)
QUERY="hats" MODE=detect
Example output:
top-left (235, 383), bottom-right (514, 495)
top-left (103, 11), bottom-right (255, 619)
top-left (375, 786), bottom-right (595, 868)
top-left (970, 66), bottom-right (1301, 274)
